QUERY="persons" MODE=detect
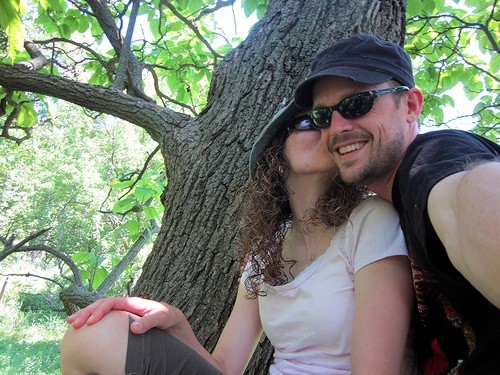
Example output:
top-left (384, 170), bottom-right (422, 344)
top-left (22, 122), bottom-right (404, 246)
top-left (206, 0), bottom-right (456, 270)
top-left (292, 34), bottom-right (500, 374)
top-left (59, 98), bottom-right (419, 374)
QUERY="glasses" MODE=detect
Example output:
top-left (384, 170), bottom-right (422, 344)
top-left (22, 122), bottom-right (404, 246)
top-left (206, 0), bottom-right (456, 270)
top-left (308, 86), bottom-right (409, 129)
top-left (287, 113), bottom-right (319, 134)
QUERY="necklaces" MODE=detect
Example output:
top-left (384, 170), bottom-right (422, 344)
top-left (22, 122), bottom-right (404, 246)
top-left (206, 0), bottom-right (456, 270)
top-left (294, 225), bottom-right (329, 261)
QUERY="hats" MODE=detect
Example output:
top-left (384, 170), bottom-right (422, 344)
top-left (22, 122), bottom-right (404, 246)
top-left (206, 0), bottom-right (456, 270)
top-left (249, 98), bottom-right (298, 182)
top-left (294, 34), bottom-right (415, 109)
